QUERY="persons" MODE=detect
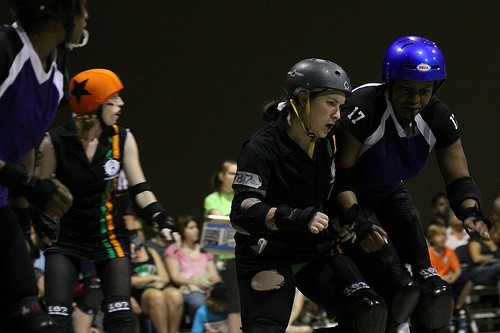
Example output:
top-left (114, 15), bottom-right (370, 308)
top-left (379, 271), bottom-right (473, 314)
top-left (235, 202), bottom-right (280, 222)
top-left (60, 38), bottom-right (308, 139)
top-left (230, 57), bottom-right (386, 333)
top-left (30, 66), bottom-right (183, 333)
top-left (1, 0), bottom-right (89, 333)
top-left (33, 160), bottom-right (500, 332)
top-left (322, 37), bottom-right (492, 333)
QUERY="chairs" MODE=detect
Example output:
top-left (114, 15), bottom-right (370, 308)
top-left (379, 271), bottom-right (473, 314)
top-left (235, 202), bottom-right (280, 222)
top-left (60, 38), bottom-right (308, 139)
top-left (455, 243), bottom-right (500, 312)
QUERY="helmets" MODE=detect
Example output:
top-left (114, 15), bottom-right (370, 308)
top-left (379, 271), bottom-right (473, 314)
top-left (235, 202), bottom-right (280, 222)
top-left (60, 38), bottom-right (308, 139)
top-left (70, 69), bottom-right (125, 116)
top-left (382, 36), bottom-right (446, 82)
top-left (285, 58), bottom-right (353, 97)
